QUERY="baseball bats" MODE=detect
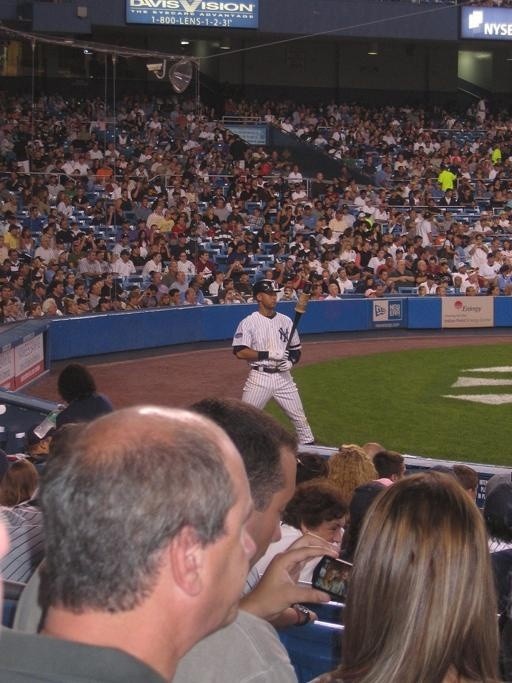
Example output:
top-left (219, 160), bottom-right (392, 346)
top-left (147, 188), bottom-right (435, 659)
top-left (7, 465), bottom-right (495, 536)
top-left (285, 280), bottom-right (313, 350)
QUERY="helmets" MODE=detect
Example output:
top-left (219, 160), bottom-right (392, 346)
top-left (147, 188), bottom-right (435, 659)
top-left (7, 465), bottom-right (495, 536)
top-left (253, 278), bottom-right (281, 293)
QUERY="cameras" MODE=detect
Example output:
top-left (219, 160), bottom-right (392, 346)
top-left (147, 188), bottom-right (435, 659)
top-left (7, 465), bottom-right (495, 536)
top-left (311, 554), bottom-right (354, 600)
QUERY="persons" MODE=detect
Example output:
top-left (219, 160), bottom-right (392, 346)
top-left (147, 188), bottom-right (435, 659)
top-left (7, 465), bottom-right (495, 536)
top-left (1, 92), bottom-right (512, 323)
top-left (230, 277), bottom-right (328, 449)
top-left (0, 359), bottom-right (512, 683)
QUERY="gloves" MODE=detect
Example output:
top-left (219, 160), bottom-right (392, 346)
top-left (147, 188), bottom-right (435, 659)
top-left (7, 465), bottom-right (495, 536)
top-left (276, 360), bottom-right (293, 371)
top-left (268, 350), bottom-right (289, 360)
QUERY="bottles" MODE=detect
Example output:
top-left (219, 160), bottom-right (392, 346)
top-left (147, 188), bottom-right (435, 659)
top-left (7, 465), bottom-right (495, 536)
top-left (33, 404), bottom-right (65, 439)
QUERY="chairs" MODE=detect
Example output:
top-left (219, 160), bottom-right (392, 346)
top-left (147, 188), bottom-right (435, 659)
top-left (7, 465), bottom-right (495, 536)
top-left (331, 105), bottom-right (508, 292)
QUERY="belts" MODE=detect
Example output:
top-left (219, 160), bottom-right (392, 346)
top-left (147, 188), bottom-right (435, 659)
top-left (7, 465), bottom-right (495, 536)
top-left (253, 366), bottom-right (279, 373)
